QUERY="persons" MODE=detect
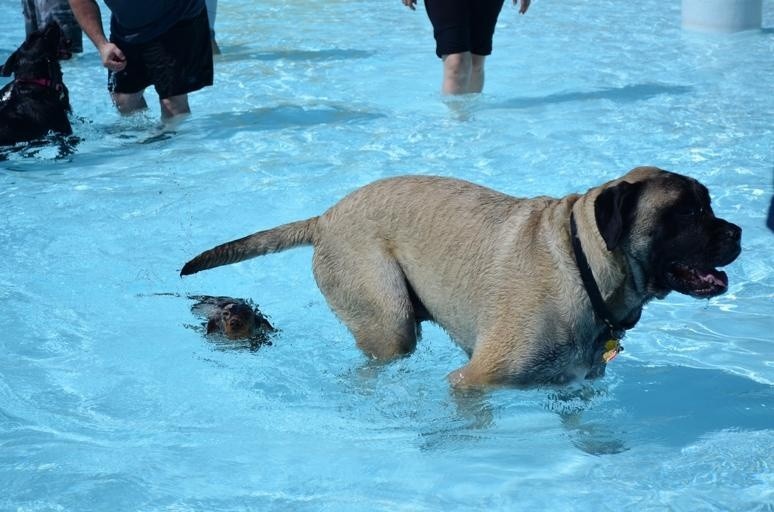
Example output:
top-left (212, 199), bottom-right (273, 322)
top-left (22, 0), bottom-right (220, 145)
top-left (402, 0), bottom-right (531, 120)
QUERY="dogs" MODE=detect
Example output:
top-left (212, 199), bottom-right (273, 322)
top-left (0, 11), bottom-right (85, 159)
top-left (179, 165), bottom-right (742, 458)
top-left (137, 292), bottom-right (281, 352)
